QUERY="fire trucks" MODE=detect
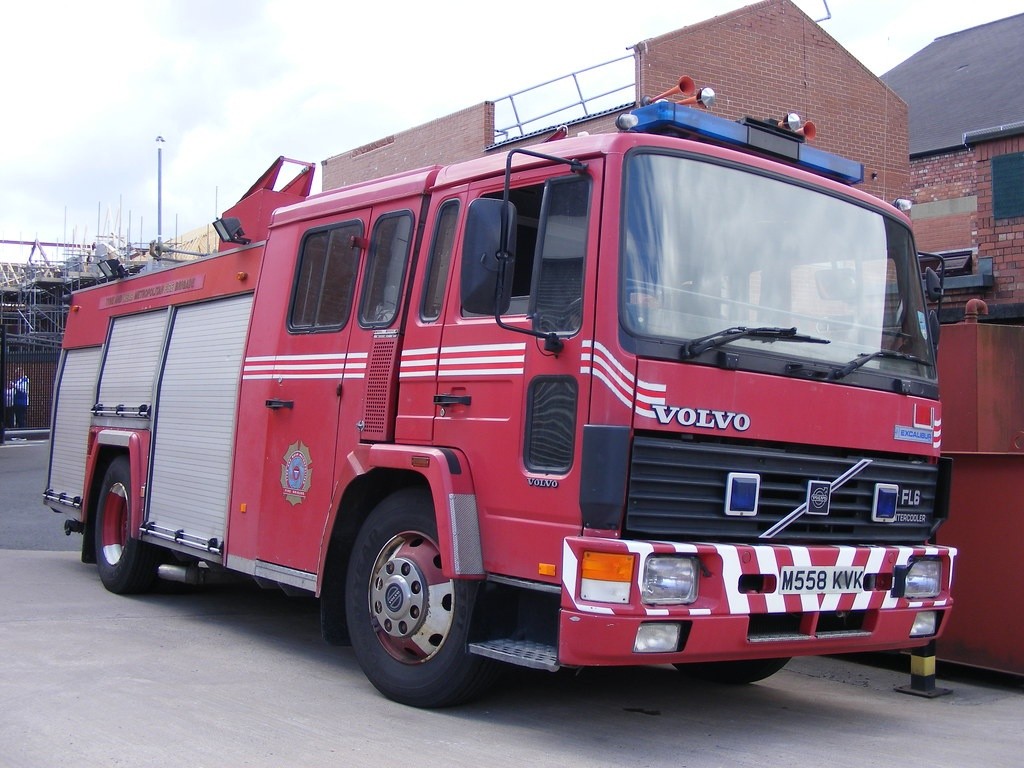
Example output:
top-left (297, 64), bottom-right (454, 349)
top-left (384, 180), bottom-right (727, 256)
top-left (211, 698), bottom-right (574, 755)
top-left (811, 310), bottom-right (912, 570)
top-left (43, 74), bottom-right (957, 708)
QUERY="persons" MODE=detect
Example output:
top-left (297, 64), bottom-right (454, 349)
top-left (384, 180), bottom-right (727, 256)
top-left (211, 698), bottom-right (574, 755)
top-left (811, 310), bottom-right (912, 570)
top-left (10, 367), bottom-right (31, 442)
top-left (5, 381), bottom-right (16, 431)
